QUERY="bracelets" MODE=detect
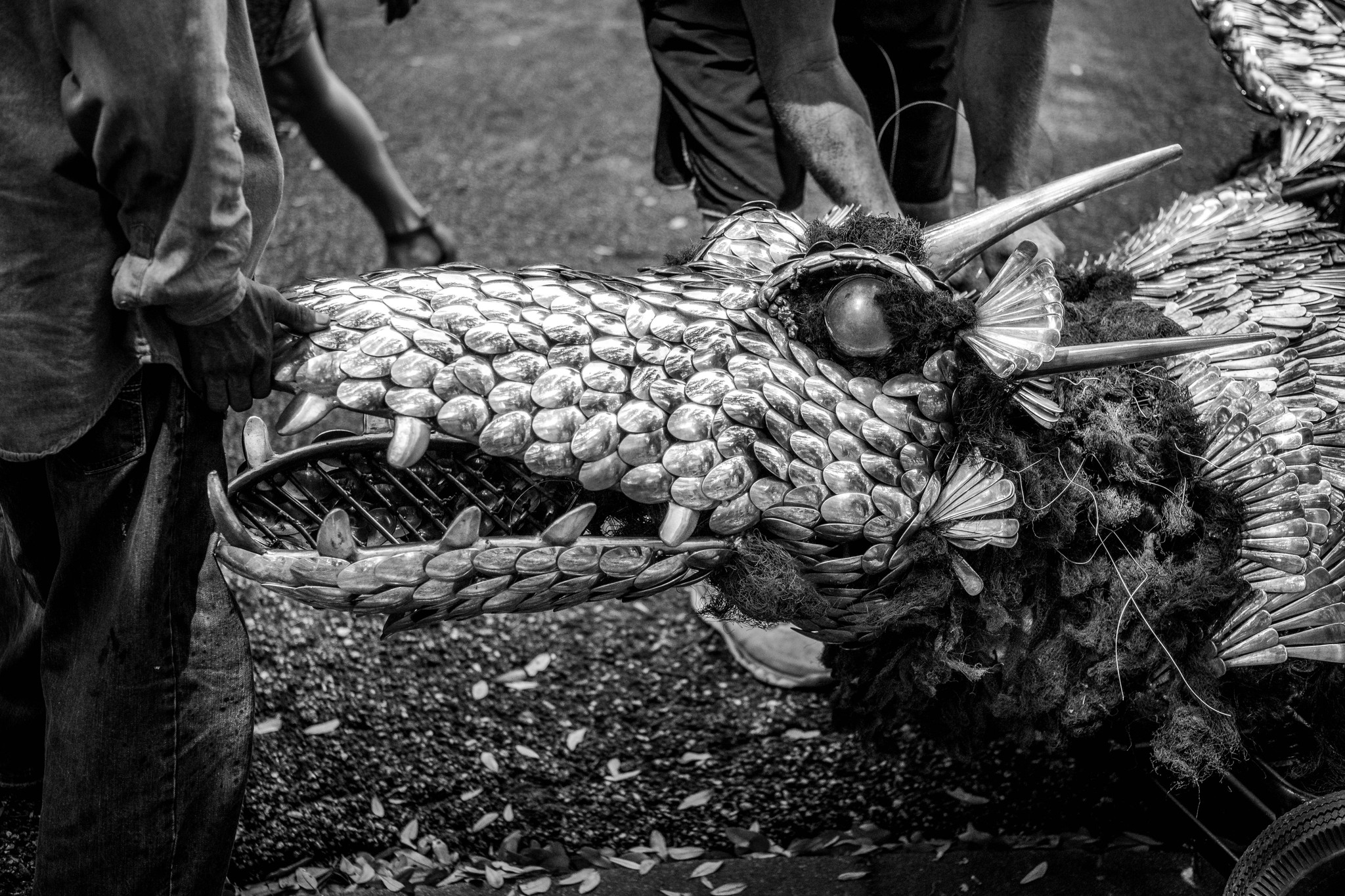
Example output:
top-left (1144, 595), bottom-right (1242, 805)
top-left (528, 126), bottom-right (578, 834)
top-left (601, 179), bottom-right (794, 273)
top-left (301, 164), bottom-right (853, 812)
top-left (384, 213), bottom-right (435, 246)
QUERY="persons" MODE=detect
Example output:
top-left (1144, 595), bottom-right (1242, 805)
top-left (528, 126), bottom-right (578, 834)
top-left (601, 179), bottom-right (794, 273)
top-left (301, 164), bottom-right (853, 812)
top-left (639, 0), bottom-right (1067, 690)
top-left (0, 0), bottom-right (459, 896)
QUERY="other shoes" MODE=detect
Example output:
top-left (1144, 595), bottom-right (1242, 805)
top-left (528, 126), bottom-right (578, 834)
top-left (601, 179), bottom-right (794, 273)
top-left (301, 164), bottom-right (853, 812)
top-left (684, 580), bottom-right (831, 688)
